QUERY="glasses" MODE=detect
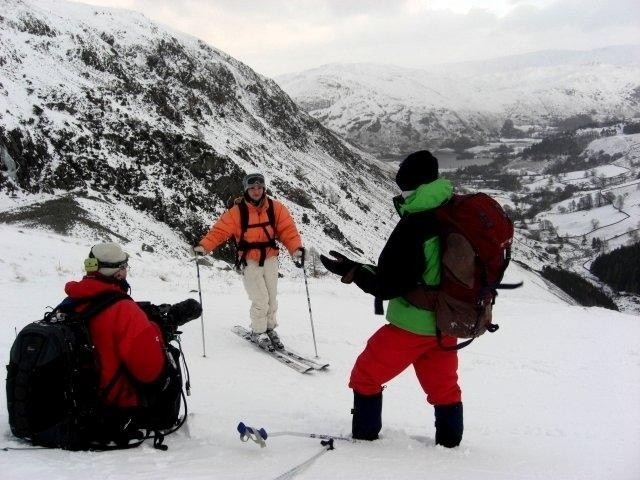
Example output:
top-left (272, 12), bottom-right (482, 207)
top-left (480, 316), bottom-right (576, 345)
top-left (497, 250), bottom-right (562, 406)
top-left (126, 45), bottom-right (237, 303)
top-left (248, 177), bottom-right (264, 184)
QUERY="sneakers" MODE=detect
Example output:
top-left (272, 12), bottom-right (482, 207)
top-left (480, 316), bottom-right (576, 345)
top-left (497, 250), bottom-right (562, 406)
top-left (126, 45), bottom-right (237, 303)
top-left (250, 332), bottom-right (275, 352)
top-left (266, 329), bottom-right (284, 350)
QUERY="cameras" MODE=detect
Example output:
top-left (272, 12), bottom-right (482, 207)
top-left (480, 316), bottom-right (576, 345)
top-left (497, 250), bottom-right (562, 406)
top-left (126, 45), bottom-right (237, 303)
top-left (138, 298), bottom-right (202, 345)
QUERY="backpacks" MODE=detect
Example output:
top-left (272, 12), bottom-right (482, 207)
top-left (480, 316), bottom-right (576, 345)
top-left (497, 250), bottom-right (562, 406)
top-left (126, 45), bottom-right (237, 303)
top-left (6, 292), bottom-right (133, 451)
top-left (415, 192), bottom-right (513, 351)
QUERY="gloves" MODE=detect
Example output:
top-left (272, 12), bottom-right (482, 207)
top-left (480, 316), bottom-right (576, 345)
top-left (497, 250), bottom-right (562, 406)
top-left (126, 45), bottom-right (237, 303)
top-left (190, 246), bottom-right (205, 257)
top-left (294, 247), bottom-right (309, 261)
top-left (320, 252), bottom-right (361, 283)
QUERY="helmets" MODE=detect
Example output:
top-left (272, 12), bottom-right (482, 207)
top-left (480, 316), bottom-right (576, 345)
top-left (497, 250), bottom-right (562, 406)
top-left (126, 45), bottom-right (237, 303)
top-left (84, 244), bottom-right (129, 276)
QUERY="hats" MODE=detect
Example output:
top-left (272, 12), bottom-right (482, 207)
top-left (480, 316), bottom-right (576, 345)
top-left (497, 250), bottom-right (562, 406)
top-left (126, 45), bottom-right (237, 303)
top-left (243, 174), bottom-right (266, 192)
top-left (396, 151), bottom-right (438, 191)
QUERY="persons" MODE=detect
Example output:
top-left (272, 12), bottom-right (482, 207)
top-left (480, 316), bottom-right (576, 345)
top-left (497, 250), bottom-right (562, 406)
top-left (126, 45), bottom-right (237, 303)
top-left (317, 148), bottom-right (464, 448)
top-left (54, 242), bottom-right (184, 436)
top-left (192, 173), bottom-right (305, 347)
